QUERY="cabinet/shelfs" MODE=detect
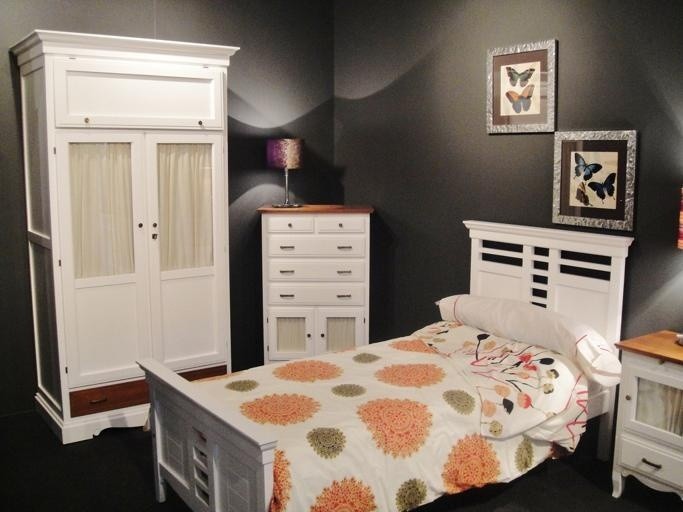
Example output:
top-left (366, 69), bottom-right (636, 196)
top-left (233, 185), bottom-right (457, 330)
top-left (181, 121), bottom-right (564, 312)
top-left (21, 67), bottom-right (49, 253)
top-left (8, 29), bottom-right (240, 445)
top-left (256, 203), bottom-right (375, 365)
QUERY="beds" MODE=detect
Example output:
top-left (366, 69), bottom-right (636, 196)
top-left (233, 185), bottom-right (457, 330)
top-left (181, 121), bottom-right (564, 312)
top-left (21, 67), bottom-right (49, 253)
top-left (136, 219), bottom-right (634, 512)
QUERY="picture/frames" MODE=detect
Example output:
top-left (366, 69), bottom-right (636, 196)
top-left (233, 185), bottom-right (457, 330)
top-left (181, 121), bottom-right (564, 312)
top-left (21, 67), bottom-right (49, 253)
top-left (552, 129), bottom-right (637, 232)
top-left (486, 39), bottom-right (556, 134)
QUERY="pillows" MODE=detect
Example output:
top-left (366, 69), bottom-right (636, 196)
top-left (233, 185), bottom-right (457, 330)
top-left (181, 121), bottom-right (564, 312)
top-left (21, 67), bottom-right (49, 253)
top-left (434, 294), bottom-right (622, 387)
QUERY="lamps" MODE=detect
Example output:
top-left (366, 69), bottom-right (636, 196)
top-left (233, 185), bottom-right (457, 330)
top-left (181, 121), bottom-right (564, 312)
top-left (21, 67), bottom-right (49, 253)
top-left (265, 137), bottom-right (306, 208)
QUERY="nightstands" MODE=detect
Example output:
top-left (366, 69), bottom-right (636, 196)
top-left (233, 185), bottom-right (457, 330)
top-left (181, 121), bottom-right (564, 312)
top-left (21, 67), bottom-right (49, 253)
top-left (611, 330), bottom-right (683, 501)
